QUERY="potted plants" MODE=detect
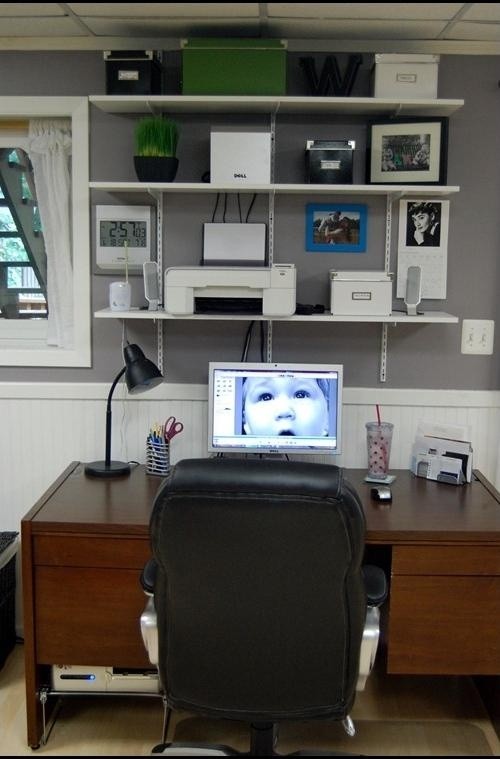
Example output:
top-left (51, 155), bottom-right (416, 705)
top-left (128, 111), bottom-right (179, 183)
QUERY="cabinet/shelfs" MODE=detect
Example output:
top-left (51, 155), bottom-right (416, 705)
top-left (17, 454), bottom-right (498, 754)
top-left (80, 88), bottom-right (468, 334)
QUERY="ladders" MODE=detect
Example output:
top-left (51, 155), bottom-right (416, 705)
top-left (0, 149), bottom-right (48, 318)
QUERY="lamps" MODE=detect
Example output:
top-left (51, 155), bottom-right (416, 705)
top-left (78, 339), bottom-right (166, 480)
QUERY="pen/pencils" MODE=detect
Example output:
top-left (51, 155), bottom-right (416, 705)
top-left (147, 425), bottom-right (165, 443)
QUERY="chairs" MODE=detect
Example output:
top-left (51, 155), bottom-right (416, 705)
top-left (135, 453), bottom-right (393, 757)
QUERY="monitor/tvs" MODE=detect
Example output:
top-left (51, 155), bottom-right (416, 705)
top-left (208, 361), bottom-right (344, 457)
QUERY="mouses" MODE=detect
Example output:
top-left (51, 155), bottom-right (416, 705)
top-left (370, 484), bottom-right (393, 504)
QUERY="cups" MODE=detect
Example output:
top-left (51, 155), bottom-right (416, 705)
top-left (109, 281), bottom-right (132, 311)
top-left (366, 422), bottom-right (395, 479)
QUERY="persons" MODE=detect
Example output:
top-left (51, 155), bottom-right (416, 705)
top-left (382, 141), bottom-right (429, 171)
top-left (242, 377), bottom-right (336, 439)
top-left (407, 203), bottom-right (440, 247)
top-left (317, 211), bottom-right (352, 244)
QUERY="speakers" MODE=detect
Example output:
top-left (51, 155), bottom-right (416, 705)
top-left (142, 261), bottom-right (161, 312)
top-left (403, 265), bottom-right (423, 316)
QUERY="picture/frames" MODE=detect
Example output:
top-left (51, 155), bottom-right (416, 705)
top-left (302, 201), bottom-right (367, 254)
top-left (362, 114), bottom-right (451, 186)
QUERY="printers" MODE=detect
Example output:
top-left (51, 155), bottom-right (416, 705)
top-left (163, 222), bottom-right (298, 316)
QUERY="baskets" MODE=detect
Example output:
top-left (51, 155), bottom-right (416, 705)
top-left (144, 439), bottom-right (170, 476)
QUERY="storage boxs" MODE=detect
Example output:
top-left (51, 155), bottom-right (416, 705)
top-left (101, 48), bottom-right (165, 96)
top-left (328, 268), bottom-right (394, 317)
top-left (367, 50), bottom-right (440, 102)
top-left (300, 138), bottom-right (357, 185)
top-left (176, 37), bottom-right (288, 100)
top-left (286, 47), bottom-right (375, 99)
top-left (208, 124), bottom-right (273, 185)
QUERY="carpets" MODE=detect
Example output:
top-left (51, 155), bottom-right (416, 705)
top-left (173, 714), bottom-right (495, 758)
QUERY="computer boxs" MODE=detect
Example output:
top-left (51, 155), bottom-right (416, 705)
top-left (51, 663), bottom-right (160, 694)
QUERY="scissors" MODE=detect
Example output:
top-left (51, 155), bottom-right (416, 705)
top-left (165, 416), bottom-right (183, 444)
